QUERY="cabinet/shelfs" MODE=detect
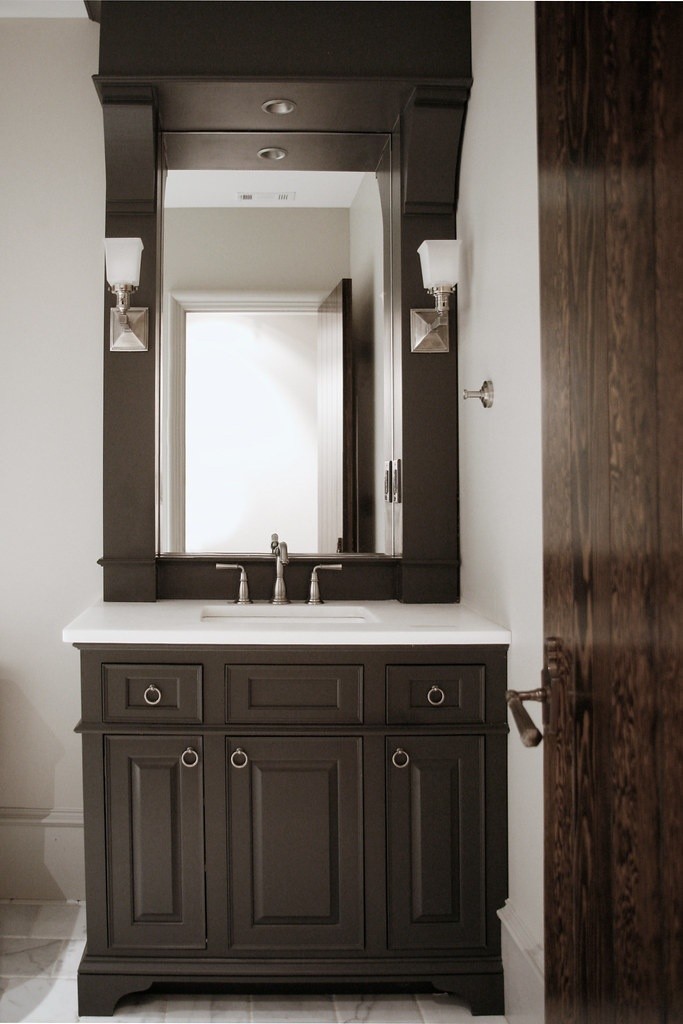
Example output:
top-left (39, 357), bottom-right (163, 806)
top-left (72, 643), bottom-right (511, 960)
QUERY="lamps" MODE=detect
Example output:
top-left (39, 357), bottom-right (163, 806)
top-left (104, 237), bottom-right (149, 353)
top-left (410, 240), bottom-right (464, 353)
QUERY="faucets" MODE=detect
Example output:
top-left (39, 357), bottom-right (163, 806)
top-left (271, 533), bottom-right (280, 552)
top-left (270, 543), bottom-right (291, 604)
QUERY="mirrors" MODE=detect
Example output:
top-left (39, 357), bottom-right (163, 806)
top-left (153, 131), bottom-right (394, 558)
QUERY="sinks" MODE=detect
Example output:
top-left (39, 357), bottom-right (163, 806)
top-left (200, 606), bottom-right (380, 622)
